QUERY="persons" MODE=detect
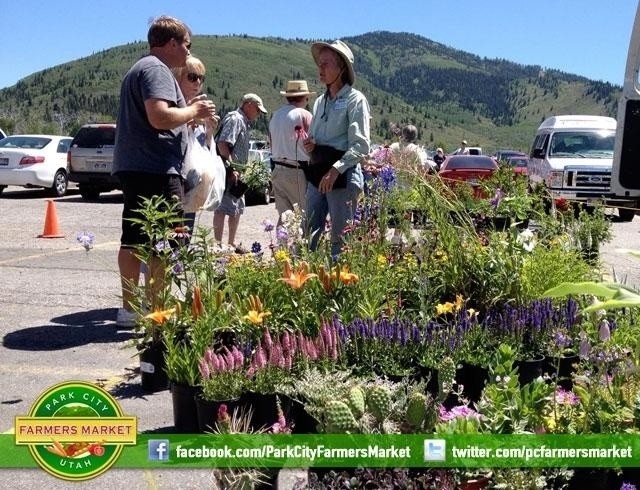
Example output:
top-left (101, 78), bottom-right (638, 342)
top-left (390, 124), bottom-right (422, 242)
top-left (112, 14), bottom-right (217, 328)
top-left (212, 93), bottom-right (268, 252)
top-left (456, 140), bottom-right (470, 156)
top-left (434, 148), bottom-right (446, 173)
top-left (170, 54), bottom-right (220, 252)
top-left (268, 81), bottom-right (316, 241)
top-left (303, 40), bottom-right (372, 256)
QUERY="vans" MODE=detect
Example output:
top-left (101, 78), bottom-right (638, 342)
top-left (526, 113), bottom-right (634, 224)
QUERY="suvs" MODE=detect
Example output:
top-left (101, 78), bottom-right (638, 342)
top-left (67, 122), bottom-right (117, 199)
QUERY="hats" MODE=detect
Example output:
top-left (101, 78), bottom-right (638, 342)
top-left (279, 81), bottom-right (317, 97)
top-left (242, 93), bottom-right (268, 114)
top-left (310, 36), bottom-right (356, 87)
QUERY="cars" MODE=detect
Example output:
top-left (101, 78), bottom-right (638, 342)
top-left (0, 129), bottom-right (7, 139)
top-left (1, 134), bottom-right (76, 196)
top-left (493, 149), bottom-right (526, 162)
top-left (246, 151), bottom-right (275, 203)
top-left (503, 156), bottom-right (529, 178)
top-left (437, 154), bottom-right (503, 198)
top-left (608, 1), bottom-right (640, 202)
top-left (246, 139), bottom-right (266, 150)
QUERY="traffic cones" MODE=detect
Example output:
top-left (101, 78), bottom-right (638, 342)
top-left (36, 198), bottom-right (68, 240)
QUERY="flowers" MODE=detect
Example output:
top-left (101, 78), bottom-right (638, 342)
top-left (115, 148), bottom-right (639, 490)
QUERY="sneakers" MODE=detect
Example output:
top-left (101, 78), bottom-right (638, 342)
top-left (116, 308), bottom-right (150, 327)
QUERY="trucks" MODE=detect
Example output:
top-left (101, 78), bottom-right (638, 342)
top-left (451, 147), bottom-right (483, 155)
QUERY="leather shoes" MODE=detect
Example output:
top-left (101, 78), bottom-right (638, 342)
top-left (235, 243), bottom-right (250, 254)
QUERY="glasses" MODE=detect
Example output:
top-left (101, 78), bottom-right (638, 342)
top-left (187, 72), bottom-right (205, 83)
top-left (182, 38), bottom-right (192, 50)
